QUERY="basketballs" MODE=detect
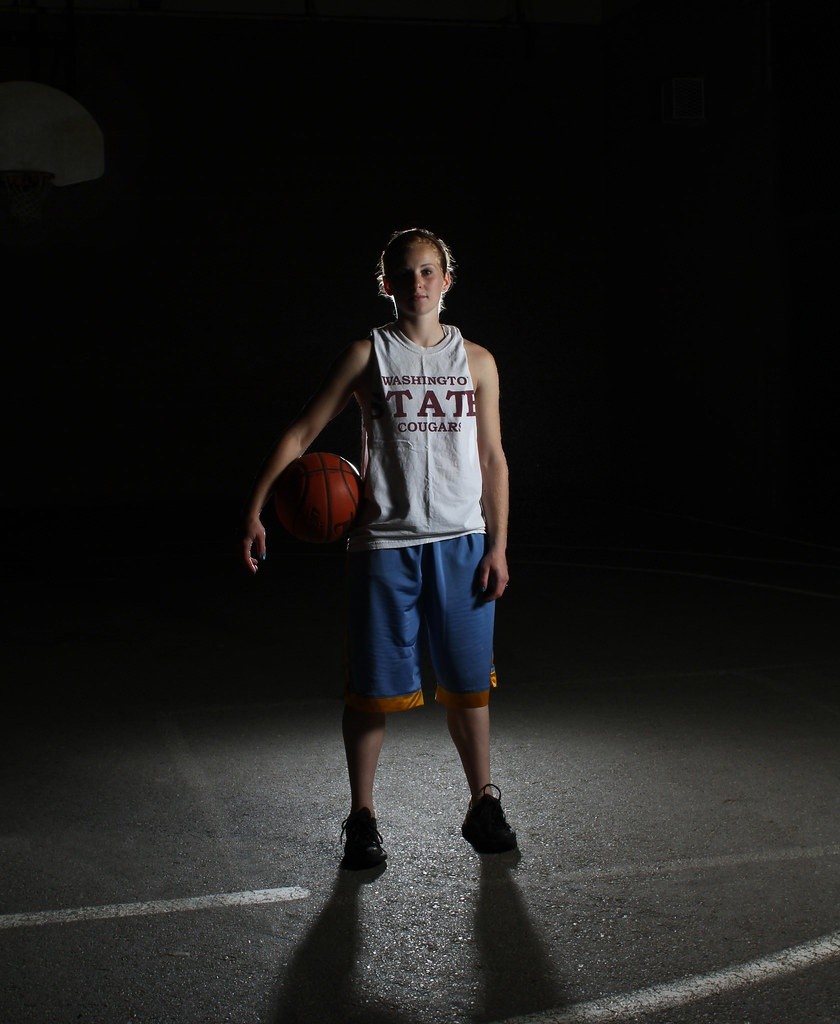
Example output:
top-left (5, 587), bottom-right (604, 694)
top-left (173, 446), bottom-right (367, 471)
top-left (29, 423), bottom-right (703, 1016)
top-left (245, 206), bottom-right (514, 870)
top-left (274, 454), bottom-right (363, 545)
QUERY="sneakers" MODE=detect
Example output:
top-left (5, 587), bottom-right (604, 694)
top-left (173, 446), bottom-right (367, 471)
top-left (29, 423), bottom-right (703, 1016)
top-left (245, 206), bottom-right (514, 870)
top-left (461, 784), bottom-right (517, 855)
top-left (339, 807), bottom-right (387, 872)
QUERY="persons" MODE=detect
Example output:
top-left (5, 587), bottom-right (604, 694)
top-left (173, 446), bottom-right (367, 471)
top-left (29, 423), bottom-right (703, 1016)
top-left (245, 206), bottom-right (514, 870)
top-left (241, 229), bottom-right (516, 870)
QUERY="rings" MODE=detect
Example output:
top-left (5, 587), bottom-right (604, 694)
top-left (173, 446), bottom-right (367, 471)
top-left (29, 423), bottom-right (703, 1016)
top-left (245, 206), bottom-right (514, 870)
top-left (505, 584), bottom-right (508, 587)
